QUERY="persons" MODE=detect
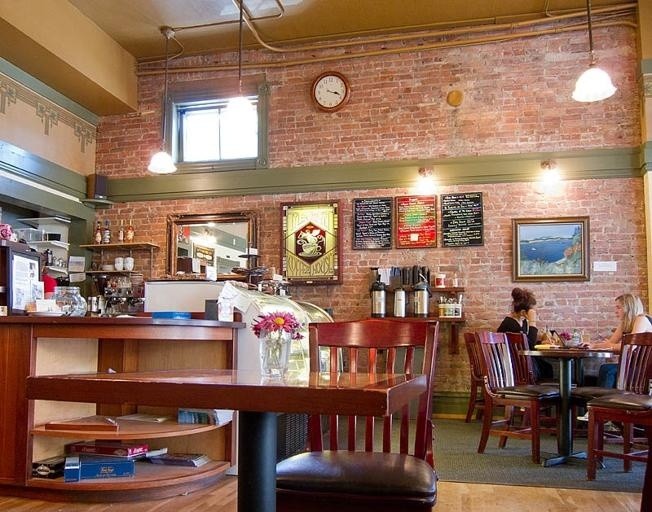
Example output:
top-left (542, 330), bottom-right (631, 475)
top-left (577, 294), bottom-right (651, 421)
top-left (497, 288), bottom-right (554, 383)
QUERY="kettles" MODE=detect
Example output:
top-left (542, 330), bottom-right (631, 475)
top-left (44, 248), bottom-right (54, 266)
top-left (368, 280), bottom-right (388, 318)
top-left (411, 273), bottom-right (432, 317)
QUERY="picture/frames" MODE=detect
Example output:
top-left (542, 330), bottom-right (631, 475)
top-left (352, 191), bottom-right (484, 251)
top-left (279, 199), bottom-right (344, 285)
top-left (511, 214), bottom-right (591, 282)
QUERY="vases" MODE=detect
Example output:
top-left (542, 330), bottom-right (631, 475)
top-left (260, 328), bottom-right (292, 378)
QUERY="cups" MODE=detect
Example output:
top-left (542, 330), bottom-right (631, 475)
top-left (393, 287), bottom-right (406, 318)
top-left (437, 303), bottom-right (463, 319)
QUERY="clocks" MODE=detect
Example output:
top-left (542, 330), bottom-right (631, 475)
top-left (309, 71), bottom-right (352, 112)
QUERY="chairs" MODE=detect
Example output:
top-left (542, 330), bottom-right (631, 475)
top-left (275, 316), bottom-right (441, 512)
top-left (463, 328), bottom-right (652, 482)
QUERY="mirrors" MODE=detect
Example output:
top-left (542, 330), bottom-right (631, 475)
top-left (165, 209), bottom-right (259, 282)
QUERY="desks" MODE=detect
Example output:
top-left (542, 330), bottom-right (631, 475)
top-left (23, 367), bottom-right (429, 511)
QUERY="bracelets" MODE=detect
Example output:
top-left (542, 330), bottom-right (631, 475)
top-left (529, 320), bottom-right (536, 322)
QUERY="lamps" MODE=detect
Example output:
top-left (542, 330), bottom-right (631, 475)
top-left (146, 25), bottom-right (178, 174)
top-left (570, 0), bottom-right (618, 103)
top-left (223, 0), bottom-right (258, 138)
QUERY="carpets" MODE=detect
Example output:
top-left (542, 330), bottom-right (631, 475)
top-left (225, 415), bottom-right (646, 493)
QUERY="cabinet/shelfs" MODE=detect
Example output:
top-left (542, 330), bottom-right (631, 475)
top-left (78, 241), bottom-right (161, 278)
top-left (17, 215), bottom-right (71, 281)
top-left (144, 279), bottom-right (345, 417)
top-left (369, 285), bottom-right (466, 355)
top-left (0, 313), bottom-right (245, 506)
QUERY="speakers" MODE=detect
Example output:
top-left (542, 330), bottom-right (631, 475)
top-left (88, 174), bottom-right (108, 200)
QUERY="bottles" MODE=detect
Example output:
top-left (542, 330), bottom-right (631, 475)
top-left (95, 219), bottom-right (135, 244)
top-left (572, 328), bottom-right (579, 346)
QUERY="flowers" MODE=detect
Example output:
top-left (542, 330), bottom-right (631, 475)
top-left (251, 310), bottom-right (307, 370)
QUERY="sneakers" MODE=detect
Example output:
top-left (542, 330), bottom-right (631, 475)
top-left (576, 410), bottom-right (590, 421)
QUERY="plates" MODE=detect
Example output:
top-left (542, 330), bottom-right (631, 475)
top-left (102, 264), bottom-right (114, 270)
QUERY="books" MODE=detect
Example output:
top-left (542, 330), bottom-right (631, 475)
top-left (178, 409), bottom-right (234, 425)
top-left (152, 454), bottom-right (212, 467)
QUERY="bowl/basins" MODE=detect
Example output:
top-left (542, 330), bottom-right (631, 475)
top-left (115, 256), bottom-right (136, 271)
